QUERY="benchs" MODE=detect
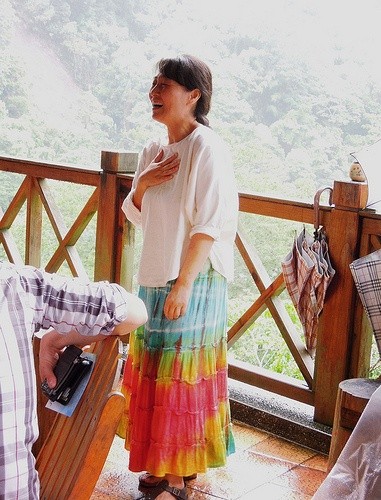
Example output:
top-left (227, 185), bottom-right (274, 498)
top-left (28, 330), bottom-right (128, 500)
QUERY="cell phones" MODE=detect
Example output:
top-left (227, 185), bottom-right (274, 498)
top-left (40, 345), bottom-right (84, 395)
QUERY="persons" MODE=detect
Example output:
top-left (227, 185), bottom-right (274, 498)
top-left (1, 261), bottom-right (150, 500)
top-left (120, 52), bottom-right (239, 500)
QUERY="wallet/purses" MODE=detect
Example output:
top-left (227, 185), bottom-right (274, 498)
top-left (40, 345), bottom-right (92, 405)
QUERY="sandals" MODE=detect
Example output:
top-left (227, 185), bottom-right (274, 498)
top-left (139, 471), bottom-right (197, 486)
top-left (154, 484), bottom-right (188, 500)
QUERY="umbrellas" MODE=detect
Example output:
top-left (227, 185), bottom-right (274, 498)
top-left (349, 247), bottom-right (381, 363)
top-left (282, 187), bottom-right (336, 359)
